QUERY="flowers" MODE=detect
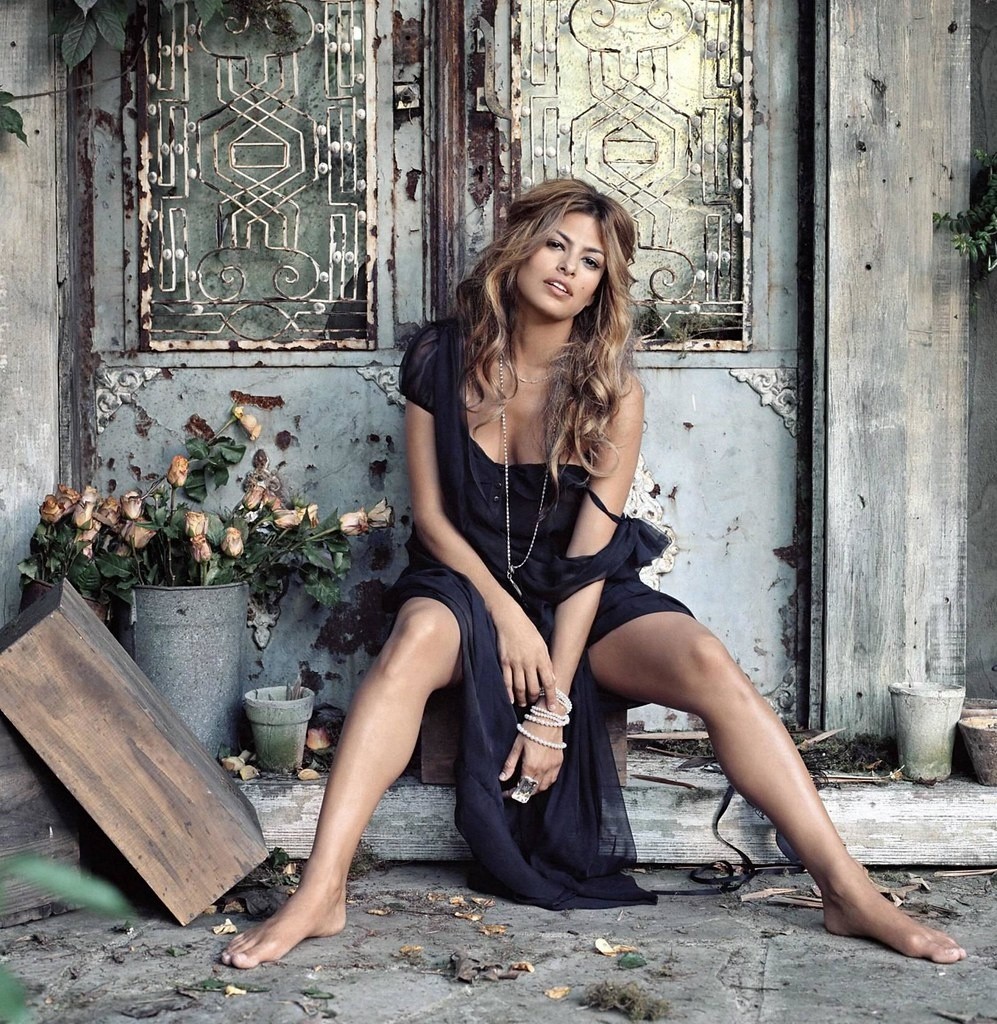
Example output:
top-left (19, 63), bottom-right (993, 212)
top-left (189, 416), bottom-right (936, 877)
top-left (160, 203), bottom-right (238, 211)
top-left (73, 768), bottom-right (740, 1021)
top-left (21, 391), bottom-right (393, 627)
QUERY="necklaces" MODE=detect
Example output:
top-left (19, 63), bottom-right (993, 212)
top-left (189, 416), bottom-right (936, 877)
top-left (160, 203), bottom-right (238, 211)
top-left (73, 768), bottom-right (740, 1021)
top-left (518, 372), bottom-right (556, 383)
top-left (499, 354), bottom-right (556, 595)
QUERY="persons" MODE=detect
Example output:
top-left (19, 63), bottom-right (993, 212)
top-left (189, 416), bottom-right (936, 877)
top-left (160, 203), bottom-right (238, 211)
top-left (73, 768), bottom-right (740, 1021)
top-left (220, 180), bottom-right (965, 968)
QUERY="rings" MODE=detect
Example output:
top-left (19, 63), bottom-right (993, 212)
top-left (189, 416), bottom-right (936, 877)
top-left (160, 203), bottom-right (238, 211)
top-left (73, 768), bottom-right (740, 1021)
top-left (511, 776), bottom-right (538, 803)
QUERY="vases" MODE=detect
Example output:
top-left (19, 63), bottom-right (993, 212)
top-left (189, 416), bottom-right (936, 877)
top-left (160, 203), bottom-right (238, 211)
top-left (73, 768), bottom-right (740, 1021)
top-left (132, 582), bottom-right (249, 777)
top-left (957, 716), bottom-right (997, 785)
top-left (244, 687), bottom-right (315, 774)
top-left (18, 576), bottom-right (109, 629)
top-left (959, 699), bottom-right (997, 717)
top-left (890, 682), bottom-right (965, 786)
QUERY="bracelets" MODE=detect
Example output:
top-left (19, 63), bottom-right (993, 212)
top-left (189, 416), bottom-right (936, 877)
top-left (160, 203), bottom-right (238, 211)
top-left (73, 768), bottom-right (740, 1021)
top-left (517, 686), bottom-right (572, 749)
